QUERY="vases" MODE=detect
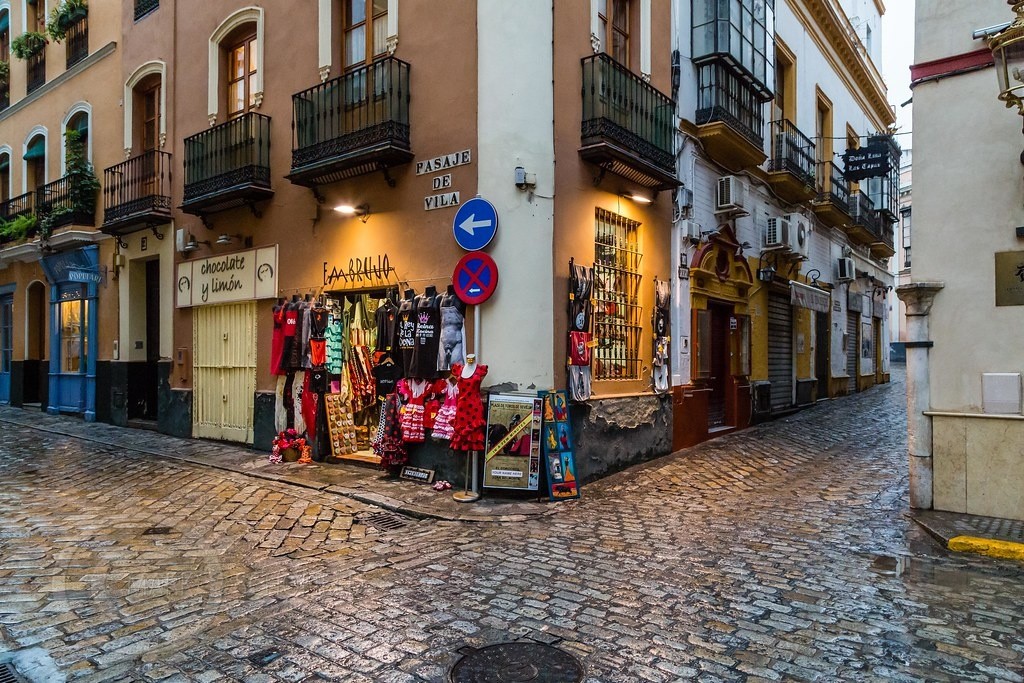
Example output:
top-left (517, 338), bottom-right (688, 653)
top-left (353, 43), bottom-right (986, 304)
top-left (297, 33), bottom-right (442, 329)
top-left (280, 447), bottom-right (301, 462)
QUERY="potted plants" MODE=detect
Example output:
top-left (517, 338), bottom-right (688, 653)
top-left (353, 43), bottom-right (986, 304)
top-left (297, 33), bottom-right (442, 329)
top-left (44, 0), bottom-right (88, 44)
top-left (10, 32), bottom-right (49, 61)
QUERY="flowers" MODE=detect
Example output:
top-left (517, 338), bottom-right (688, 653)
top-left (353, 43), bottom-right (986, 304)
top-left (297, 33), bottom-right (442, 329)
top-left (269, 428), bottom-right (313, 464)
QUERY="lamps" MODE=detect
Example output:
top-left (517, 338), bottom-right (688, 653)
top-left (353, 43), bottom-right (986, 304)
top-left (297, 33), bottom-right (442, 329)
top-left (185, 240), bottom-right (210, 251)
top-left (757, 252), bottom-right (778, 282)
top-left (215, 234), bottom-right (241, 245)
top-left (805, 268), bottom-right (820, 288)
top-left (986, 0), bottom-right (1024, 164)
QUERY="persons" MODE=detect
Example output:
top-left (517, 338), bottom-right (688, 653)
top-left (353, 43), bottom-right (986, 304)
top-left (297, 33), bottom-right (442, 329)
top-left (448, 354), bottom-right (488, 451)
top-left (270, 293), bottom-right (314, 374)
top-left (393, 284), bottom-right (466, 376)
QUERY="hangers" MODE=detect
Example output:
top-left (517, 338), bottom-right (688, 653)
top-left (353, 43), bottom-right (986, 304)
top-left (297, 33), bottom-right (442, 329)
top-left (380, 353), bottom-right (395, 366)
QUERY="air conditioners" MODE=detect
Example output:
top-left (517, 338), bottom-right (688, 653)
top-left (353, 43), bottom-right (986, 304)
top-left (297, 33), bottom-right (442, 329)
top-left (714, 175), bottom-right (751, 218)
top-left (783, 212), bottom-right (809, 262)
top-left (759, 216), bottom-right (792, 255)
top-left (834, 257), bottom-right (855, 285)
